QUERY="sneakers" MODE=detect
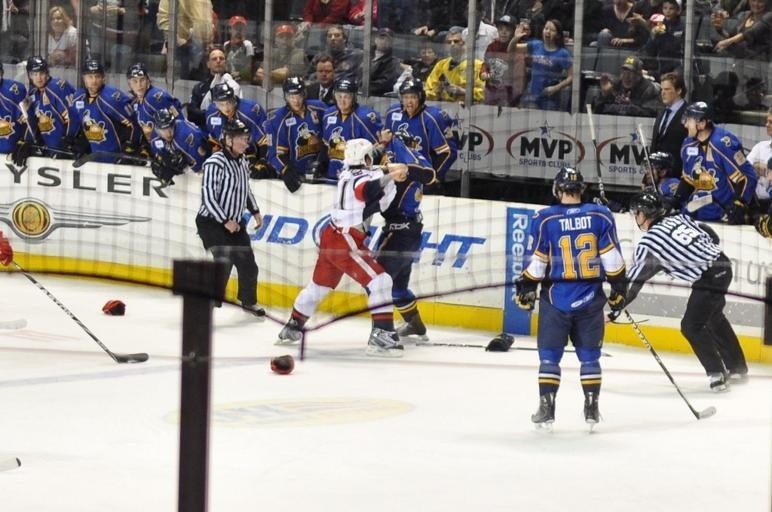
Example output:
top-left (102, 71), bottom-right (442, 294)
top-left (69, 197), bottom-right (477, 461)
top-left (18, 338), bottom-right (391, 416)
top-left (531, 391), bottom-right (557, 422)
top-left (273, 326), bottom-right (304, 341)
top-left (243, 304), bottom-right (265, 317)
top-left (584, 391), bottom-right (599, 422)
top-left (710, 373), bottom-right (727, 388)
top-left (366, 327), bottom-right (403, 350)
top-left (728, 369), bottom-right (749, 377)
top-left (397, 321), bottom-right (427, 337)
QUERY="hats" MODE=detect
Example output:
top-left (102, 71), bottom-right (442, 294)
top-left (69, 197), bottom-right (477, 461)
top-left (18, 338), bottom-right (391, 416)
top-left (276, 26), bottom-right (294, 36)
top-left (229, 16), bottom-right (247, 25)
top-left (379, 28), bottom-right (394, 40)
top-left (622, 57), bottom-right (643, 72)
top-left (495, 15), bottom-right (517, 29)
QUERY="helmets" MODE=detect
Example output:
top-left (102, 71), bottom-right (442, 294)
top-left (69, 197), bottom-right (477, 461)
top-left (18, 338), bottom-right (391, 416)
top-left (681, 101), bottom-right (709, 128)
top-left (344, 138), bottom-right (373, 169)
top-left (282, 76), bottom-right (304, 96)
top-left (126, 64), bottom-right (150, 80)
top-left (333, 80), bottom-right (358, 95)
top-left (630, 194), bottom-right (665, 218)
top-left (212, 83), bottom-right (235, 103)
top-left (552, 168), bottom-right (584, 197)
top-left (155, 110), bottom-right (175, 139)
top-left (81, 61), bottom-right (104, 74)
top-left (26, 56), bottom-right (49, 72)
top-left (640, 152), bottom-right (673, 174)
top-left (399, 78), bottom-right (425, 95)
top-left (220, 119), bottom-right (251, 154)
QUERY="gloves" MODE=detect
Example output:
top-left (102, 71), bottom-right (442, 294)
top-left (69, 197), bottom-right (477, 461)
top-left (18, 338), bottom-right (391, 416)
top-left (283, 169), bottom-right (304, 193)
top-left (721, 198), bottom-right (746, 225)
top-left (608, 290), bottom-right (626, 310)
top-left (0, 231), bottom-right (12, 266)
top-left (514, 275), bottom-right (539, 309)
top-left (753, 214), bottom-right (772, 239)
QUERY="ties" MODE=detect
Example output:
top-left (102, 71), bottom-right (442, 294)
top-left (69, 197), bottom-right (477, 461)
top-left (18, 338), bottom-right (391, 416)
top-left (660, 108), bottom-right (672, 136)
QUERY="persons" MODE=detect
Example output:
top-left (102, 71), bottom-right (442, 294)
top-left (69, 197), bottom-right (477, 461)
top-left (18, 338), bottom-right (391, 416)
top-left (278, 139), bottom-right (406, 352)
top-left (2, 1), bottom-right (772, 237)
top-left (364, 130), bottom-right (437, 335)
top-left (514, 167), bottom-right (628, 426)
top-left (196, 120), bottom-right (267, 318)
top-left (603, 192), bottom-right (749, 389)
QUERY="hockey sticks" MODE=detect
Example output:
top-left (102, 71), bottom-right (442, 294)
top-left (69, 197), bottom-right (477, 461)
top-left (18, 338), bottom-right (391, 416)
top-left (608, 308), bottom-right (648, 325)
top-left (10, 259), bottom-right (148, 365)
top-left (622, 308), bottom-right (716, 420)
top-left (72, 150), bottom-right (150, 168)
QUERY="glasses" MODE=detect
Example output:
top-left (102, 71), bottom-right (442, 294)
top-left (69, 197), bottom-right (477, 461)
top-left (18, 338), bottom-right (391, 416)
top-left (446, 40), bottom-right (461, 44)
